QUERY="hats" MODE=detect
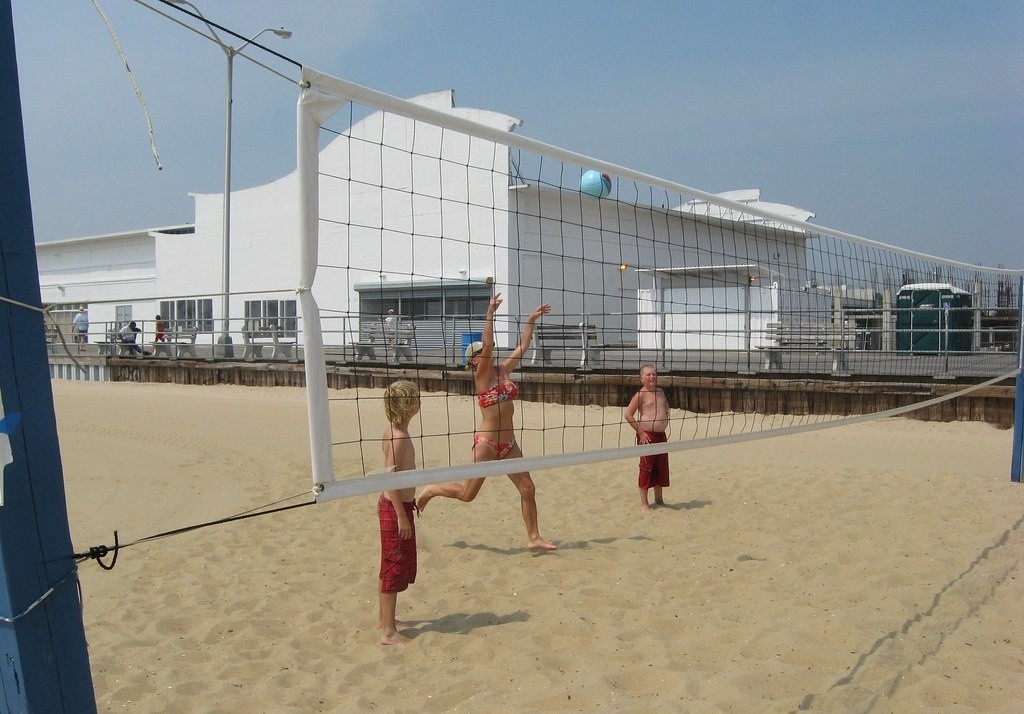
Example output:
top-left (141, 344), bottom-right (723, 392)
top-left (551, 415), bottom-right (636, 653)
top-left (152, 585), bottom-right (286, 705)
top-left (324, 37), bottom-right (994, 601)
top-left (465, 341), bottom-right (495, 363)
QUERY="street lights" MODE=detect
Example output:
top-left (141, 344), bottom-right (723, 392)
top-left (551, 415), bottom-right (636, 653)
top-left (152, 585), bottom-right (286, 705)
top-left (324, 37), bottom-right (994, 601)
top-left (157, 0), bottom-right (294, 357)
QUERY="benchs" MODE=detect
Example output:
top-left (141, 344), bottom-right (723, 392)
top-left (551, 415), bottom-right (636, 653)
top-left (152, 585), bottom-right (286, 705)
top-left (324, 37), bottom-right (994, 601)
top-left (530, 322), bottom-right (610, 366)
top-left (149, 326), bottom-right (198, 356)
top-left (241, 324), bottom-right (296, 358)
top-left (756, 323), bottom-right (857, 372)
top-left (348, 322), bottom-right (416, 362)
top-left (94, 329), bottom-right (137, 355)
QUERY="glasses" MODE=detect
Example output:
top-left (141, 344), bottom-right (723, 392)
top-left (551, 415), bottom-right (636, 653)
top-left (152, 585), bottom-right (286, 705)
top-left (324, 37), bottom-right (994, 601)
top-left (472, 349), bottom-right (482, 357)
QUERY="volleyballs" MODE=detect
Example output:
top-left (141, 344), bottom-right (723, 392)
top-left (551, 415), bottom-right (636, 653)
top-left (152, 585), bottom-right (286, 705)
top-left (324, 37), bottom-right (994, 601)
top-left (578, 169), bottom-right (612, 200)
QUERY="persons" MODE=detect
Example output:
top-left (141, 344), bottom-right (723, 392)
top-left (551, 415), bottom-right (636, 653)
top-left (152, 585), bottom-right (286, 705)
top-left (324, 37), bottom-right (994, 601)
top-left (154, 315), bottom-right (165, 342)
top-left (376, 381), bottom-right (421, 644)
top-left (415, 293), bottom-right (558, 549)
top-left (119, 321), bottom-right (151, 355)
top-left (625, 364), bottom-right (670, 511)
top-left (378, 309), bottom-right (397, 350)
top-left (72, 307), bottom-right (89, 343)
